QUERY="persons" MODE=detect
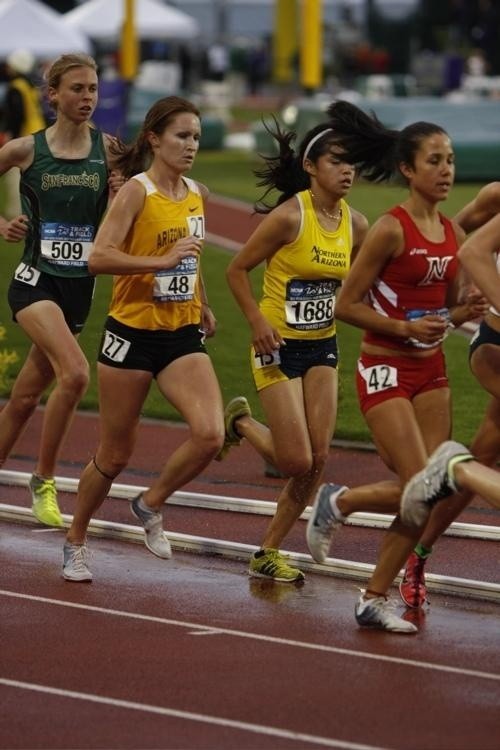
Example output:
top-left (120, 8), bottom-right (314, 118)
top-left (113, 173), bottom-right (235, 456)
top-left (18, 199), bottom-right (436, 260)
top-left (400, 212), bottom-right (500, 529)
top-left (208, 111), bottom-right (370, 582)
top-left (60, 96), bottom-right (226, 581)
top-left (398, 180), bottom-right (499, 608)
top-left (306, 100), bottom-right (489, 637)
top-left (451, 183), bottom-right (498, 228)
top-left (0, 52), bottom-right (129, 529)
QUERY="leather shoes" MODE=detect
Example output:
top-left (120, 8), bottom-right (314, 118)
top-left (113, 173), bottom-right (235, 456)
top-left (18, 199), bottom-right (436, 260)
top-left (399, 547), bottom-right (428, 609)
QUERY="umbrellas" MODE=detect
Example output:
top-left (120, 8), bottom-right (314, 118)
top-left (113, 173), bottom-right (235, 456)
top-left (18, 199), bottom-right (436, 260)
top-left (0, 0), bottom-right (95, 63)
top-left (63, 0), bottom-right (201, 42)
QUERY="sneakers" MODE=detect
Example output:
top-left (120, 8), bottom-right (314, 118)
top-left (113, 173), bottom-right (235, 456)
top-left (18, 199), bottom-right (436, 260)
top-left (400, 438), bottom-right (471, 531)
top-left (305, 482), bottom-right (350, 564)
top-left (354, 594), bottom-right (418, 634)
top-left (213, 395), bottom-right (252, 462)
top-left (129, 491), bottom-right (173, 561)
top-left (28, 473), bottom-right (65, 528)
top-left (60, 539), bottom-right (94, 582)
top-left (248, 549), bottom-right (306, 583)
